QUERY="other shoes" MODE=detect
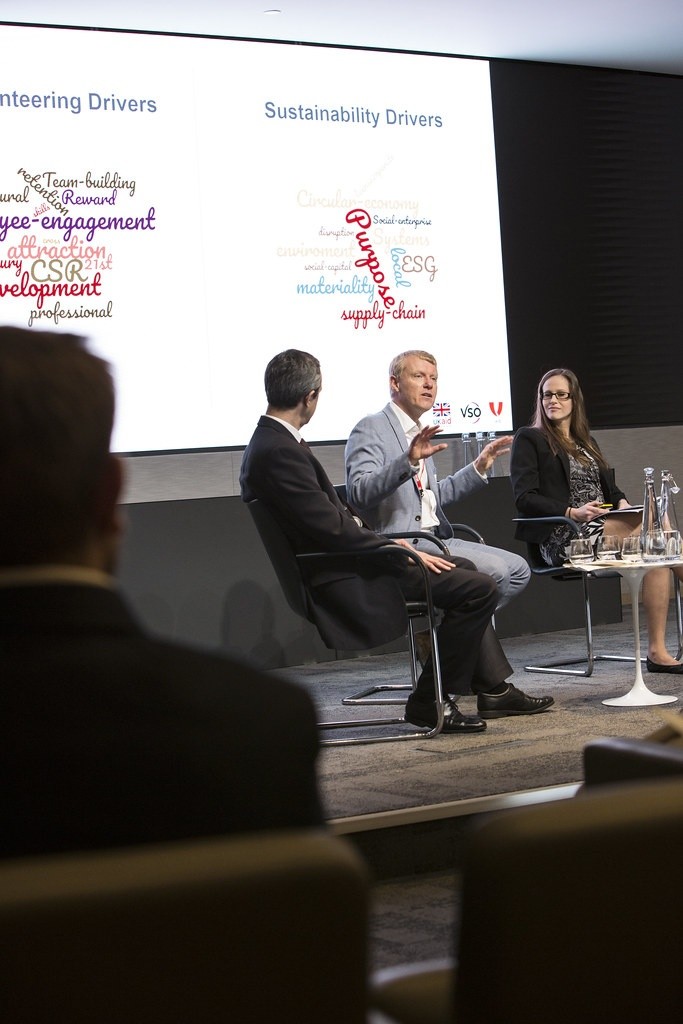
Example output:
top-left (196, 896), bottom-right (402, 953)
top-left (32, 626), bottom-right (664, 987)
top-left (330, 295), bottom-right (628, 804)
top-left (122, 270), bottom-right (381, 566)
top-left (415, 627), bottom-right (432, 669)
top-left (647, 656), bottom-right (683, 674)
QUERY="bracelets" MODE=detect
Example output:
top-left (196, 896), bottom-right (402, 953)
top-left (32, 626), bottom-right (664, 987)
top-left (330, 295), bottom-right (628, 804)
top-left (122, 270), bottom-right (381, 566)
top-left (568, 507), bottom-right (572, 520)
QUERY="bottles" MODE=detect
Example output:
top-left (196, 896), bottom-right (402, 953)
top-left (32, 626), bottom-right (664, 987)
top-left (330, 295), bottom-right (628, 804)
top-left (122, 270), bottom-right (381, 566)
top-left (474, 431), bottom-right (492, 478)
top-left (485, 431), bottom-right (505, 477)
top-left (642, 467), bottom-right (665, 564)
top-left (656, 470), bottom-right (682, 561)
top-left (461, 432), bottom-right (475, 469)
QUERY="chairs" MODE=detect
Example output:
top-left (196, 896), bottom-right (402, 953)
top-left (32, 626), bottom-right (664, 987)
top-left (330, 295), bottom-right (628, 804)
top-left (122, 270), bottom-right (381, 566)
top-left (0, 831), bottom-right (373, 1024)
top-left (451, 774), bottom-right (683, 1024)
top-left (333, 482), bottom-right (498, 705)
top-left (511, 467), bottom-right (682, 677)
top-left (245, 497), bottom-right (452, 746)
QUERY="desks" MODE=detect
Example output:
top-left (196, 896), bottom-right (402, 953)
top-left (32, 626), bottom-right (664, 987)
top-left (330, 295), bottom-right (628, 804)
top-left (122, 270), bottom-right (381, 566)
top-left (560, 553), bottom-right (682, 708)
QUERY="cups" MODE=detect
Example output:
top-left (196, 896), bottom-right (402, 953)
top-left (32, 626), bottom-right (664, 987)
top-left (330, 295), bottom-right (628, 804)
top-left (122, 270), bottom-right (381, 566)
top-left (644, 528), bottom-right (665, 557)
top-left (621, 535), bottom-right (643, 565)
top-left (596, 535), bottom-right (621, 562)
top-left (570, 533), bottom-right (595, 566)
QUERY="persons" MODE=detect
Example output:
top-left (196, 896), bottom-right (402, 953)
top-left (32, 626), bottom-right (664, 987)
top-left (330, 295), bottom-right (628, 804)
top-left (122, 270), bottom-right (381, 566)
top-left (238, 347), bottom-right (554, 737)
top-left (344, 350), bottom-right (532, 671)
top-left (0, 323), bottom-right (331, 868)
top-left (509, 368), bottom-right (683, 675)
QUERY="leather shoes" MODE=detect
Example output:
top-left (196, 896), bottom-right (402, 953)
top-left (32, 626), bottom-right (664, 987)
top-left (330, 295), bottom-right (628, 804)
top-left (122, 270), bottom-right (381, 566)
top-left (477, 682), bottom-right (555, 718)
top-left (404, 691), bottom-right (488, 733)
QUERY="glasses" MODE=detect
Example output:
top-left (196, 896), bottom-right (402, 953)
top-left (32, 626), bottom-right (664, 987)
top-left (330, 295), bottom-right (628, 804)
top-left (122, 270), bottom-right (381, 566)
top-left (540, 392), bottom-right (570, 400)
